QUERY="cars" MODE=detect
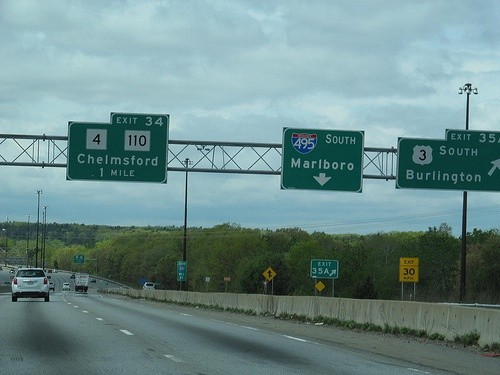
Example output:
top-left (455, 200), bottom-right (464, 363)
top-left (18, 265), bottom-right (22, 268)
top-left (53, 269), bottom-right (57, 273)
top-left (3, 281), bottom-right (11, 286)
top-left (143, 282), bottom-right (155, 290)
top-left (49, 281), bottom-right (55, 291)
top-left (48, 270), bottom-right (52, 273)
top-left (63, 283), bottom-right (70, 291)
top-left (12, 268), bottom-right (49, 302)
top-left (91, 278), bottom-right (96, 283)
top-left (70, 274), bottom-right (75, 279)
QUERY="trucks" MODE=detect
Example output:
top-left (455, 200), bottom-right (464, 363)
top-left (75, 273), bottom-right (89, 292)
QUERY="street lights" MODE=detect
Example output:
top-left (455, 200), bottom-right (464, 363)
top-left (180, 158), bottom-right (193, 290)
top-left (4, 216), bottom-right (9, 267)
top-left (25, 214), bottom-right (31, 268)
top-left (458, 83), bottom-right (478, 303)
top-left (40, 211), bottom-right (45, 268)
top-left (35, 190), bottom-right (42, 268)
top-left (43, 205), bottom-right (48, 270)
